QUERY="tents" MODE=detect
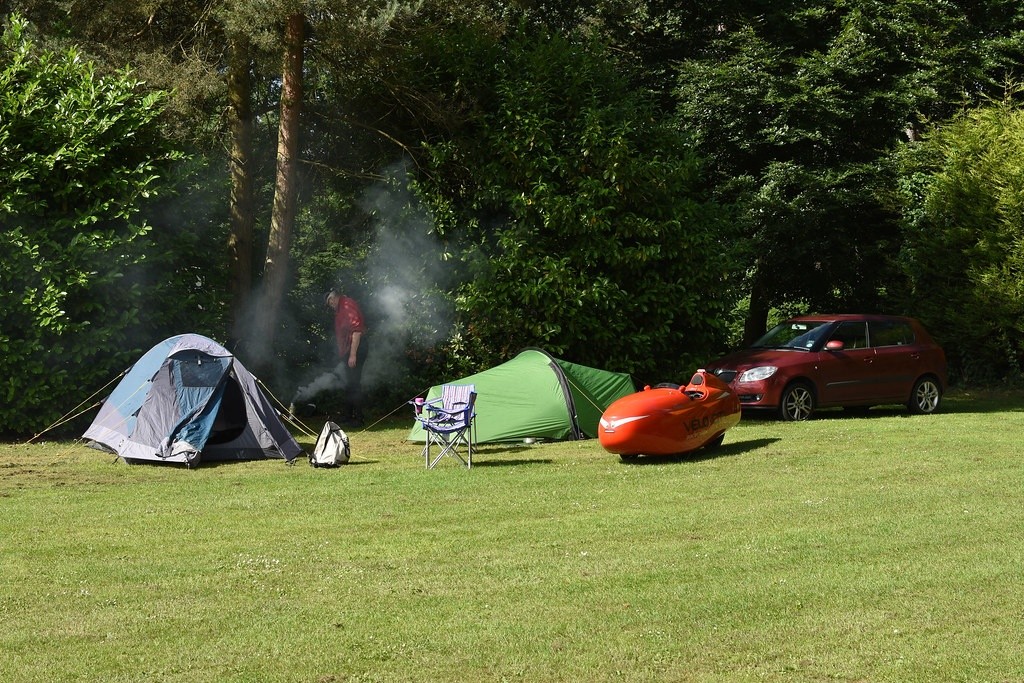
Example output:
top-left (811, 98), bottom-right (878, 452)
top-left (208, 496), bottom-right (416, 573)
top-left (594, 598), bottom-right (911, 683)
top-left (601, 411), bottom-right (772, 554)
top-left (81, 334), bottom-right (304, 466)
top-left (406, 348), bottom-right (637, 445)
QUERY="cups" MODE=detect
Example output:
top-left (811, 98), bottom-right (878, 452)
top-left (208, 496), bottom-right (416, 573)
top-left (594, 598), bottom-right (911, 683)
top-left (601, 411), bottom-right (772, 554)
top-left (415, 397), bottom-right (423, 413)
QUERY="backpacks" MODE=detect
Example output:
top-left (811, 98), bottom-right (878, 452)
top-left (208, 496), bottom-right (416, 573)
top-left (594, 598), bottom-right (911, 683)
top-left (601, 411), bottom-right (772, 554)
top-left (308, 415), bottom-right (350, 468)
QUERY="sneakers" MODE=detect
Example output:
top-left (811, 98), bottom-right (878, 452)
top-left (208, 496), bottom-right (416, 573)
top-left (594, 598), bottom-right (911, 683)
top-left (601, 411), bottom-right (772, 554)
top-left (334, 414), bottom-right (353, 424)
top-left (349, 418), bottom-right (364, 427)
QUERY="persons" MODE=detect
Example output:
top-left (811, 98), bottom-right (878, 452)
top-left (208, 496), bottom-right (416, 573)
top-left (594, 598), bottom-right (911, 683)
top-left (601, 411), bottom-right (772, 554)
top-left (323, 286), bottom-right (368, 429)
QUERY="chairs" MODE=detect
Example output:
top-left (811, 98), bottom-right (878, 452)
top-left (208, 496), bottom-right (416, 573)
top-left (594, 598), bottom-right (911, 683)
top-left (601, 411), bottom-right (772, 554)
top-left (408, 384), bottom-right (476, 471)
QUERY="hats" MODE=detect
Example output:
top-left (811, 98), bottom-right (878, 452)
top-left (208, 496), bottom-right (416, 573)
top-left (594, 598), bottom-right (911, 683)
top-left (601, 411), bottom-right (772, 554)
top-left (320, 288), bottom-right (335, 305)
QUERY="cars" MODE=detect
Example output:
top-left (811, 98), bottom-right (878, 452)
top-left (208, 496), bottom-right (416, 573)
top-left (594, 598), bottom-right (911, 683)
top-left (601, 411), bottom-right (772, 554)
top-left (704, 313), bottom-right (949, 421)
